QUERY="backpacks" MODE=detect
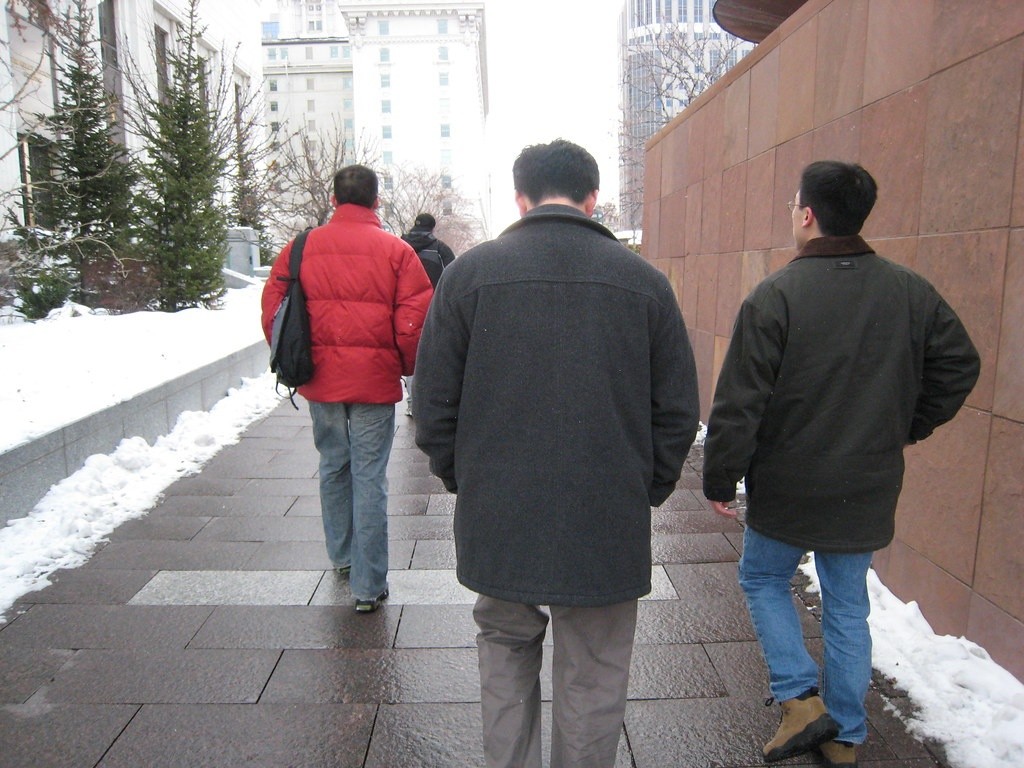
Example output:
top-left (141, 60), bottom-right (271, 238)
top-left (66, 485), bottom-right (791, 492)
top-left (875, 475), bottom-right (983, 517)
top-left (269, 226), bottom-right (312, 388)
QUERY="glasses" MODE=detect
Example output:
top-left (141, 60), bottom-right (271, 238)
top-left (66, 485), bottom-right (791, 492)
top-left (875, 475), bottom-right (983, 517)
top-left (788, 201), bottom-right (816, 215)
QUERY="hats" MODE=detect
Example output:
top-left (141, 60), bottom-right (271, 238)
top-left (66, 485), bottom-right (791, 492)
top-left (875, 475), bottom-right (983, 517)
top-left (415, 213), bottom-right (435, 228)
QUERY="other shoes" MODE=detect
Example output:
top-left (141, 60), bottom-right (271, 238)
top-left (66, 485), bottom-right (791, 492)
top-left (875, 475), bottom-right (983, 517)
top-left (336, 566), bottom-right (351, 574)
top-left (355, 587), bottom-right (389, 613)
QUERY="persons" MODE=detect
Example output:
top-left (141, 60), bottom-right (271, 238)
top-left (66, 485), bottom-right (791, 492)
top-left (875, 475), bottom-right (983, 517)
top-left (414, 141), bottom-right (701, 768)
top-left (401, 213), bottom-right (457, 416)
top-left (261, 165), bottom-right (432, 611)
top-left (705, 161), bottom-right (983, 767)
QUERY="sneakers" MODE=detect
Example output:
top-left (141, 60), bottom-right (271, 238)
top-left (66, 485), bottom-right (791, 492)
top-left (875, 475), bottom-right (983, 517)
top-left (762, 686), bottom-right (839, 764)
top-left (818, 740), bottom-right (858, 768)
